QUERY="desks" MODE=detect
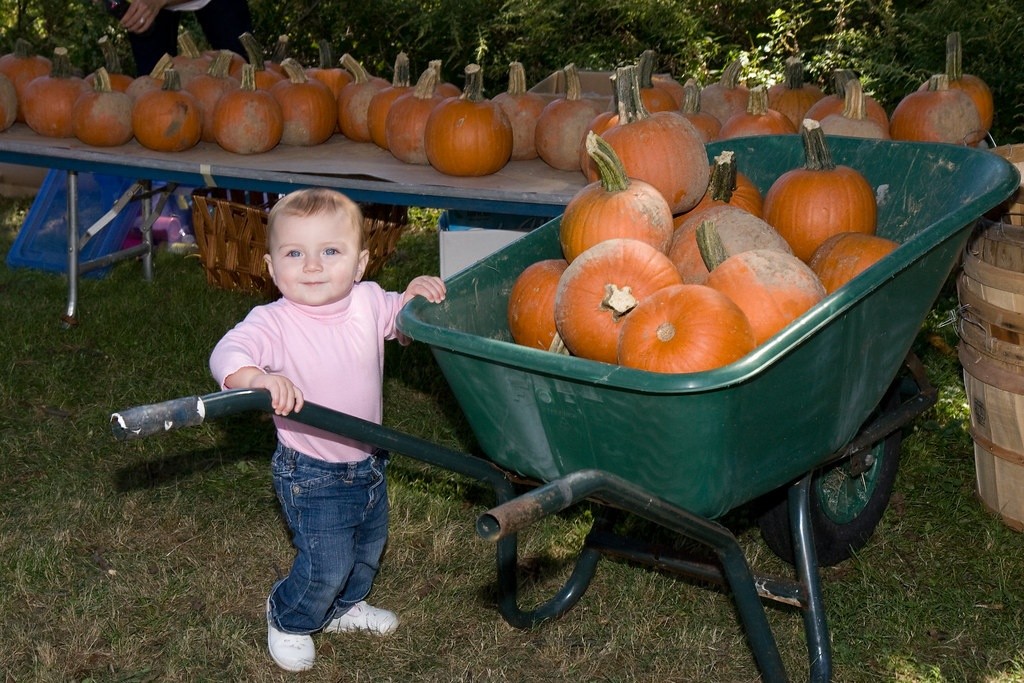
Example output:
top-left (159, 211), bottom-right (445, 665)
top-left (0, 121), bottom-right (588, 332)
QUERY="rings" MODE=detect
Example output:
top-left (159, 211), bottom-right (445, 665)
top-left (139, 17), bottom-right (145, 23)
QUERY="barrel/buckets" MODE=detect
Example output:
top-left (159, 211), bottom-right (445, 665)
top-left (956, 142), bottom-right (1024, 531)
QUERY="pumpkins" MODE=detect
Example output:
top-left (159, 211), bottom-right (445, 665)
top-left (0, 31), bottom-right (994, 176)
top-left (507, 66), bottom-right (902, 374)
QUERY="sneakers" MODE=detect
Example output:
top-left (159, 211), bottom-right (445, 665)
top-left (266, 594), bottom-right (316, 671)
top-left (321, 599), bottom-right (398, 638)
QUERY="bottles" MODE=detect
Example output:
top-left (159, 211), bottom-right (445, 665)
top-left (130, 216), bottom-right (181, 243)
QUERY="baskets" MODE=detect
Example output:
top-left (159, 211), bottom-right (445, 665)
top-left (185, 187), bottom-right (409, 299)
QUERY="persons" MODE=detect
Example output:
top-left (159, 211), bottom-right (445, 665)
top-left (209, 189), bottom-right (447, 671)
top-left (104, 0), bottom-right (254, 77)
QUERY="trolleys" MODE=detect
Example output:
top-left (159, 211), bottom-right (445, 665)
top-left (108, 135), bottom-right (1022, 683)
